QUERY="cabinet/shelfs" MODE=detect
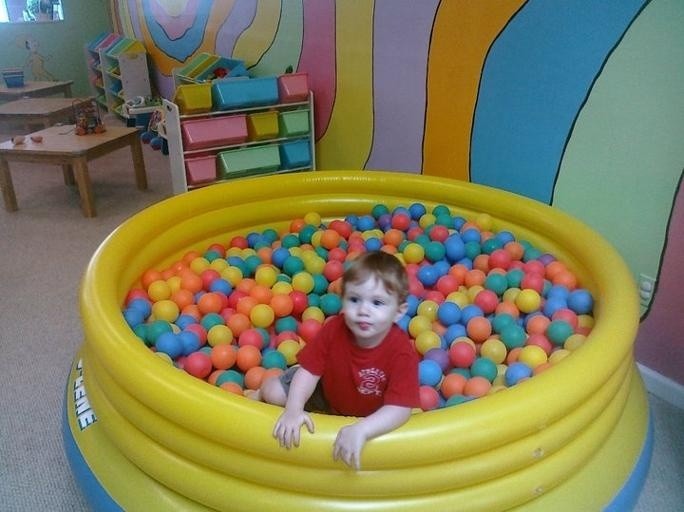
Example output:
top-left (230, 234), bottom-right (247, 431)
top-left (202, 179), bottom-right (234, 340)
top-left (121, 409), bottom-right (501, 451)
top-left (85, 44), bottom-right (151, 114)
top-left (161, 68), bottom-right (315, 196)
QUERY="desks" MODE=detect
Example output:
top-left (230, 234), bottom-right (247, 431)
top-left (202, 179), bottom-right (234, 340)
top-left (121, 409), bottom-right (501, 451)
top-left (0, 80), bottom-right (147, 214)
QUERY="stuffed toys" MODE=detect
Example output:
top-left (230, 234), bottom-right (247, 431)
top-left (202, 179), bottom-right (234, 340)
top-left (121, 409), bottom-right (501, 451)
top-left (72, 99), bottom-right (106, 135)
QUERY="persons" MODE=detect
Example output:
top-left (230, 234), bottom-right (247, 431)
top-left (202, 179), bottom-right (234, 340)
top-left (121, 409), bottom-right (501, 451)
top-left (273, 250), bottom-right (420, 468)
top-left (25, 39), bottom-right (55, 82)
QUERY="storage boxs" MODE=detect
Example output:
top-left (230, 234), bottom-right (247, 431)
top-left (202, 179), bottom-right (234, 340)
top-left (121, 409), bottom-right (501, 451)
top-left (87, 30), bottom-right (146, 113)
top-left (176, 51), bottom-right (246, 84)
